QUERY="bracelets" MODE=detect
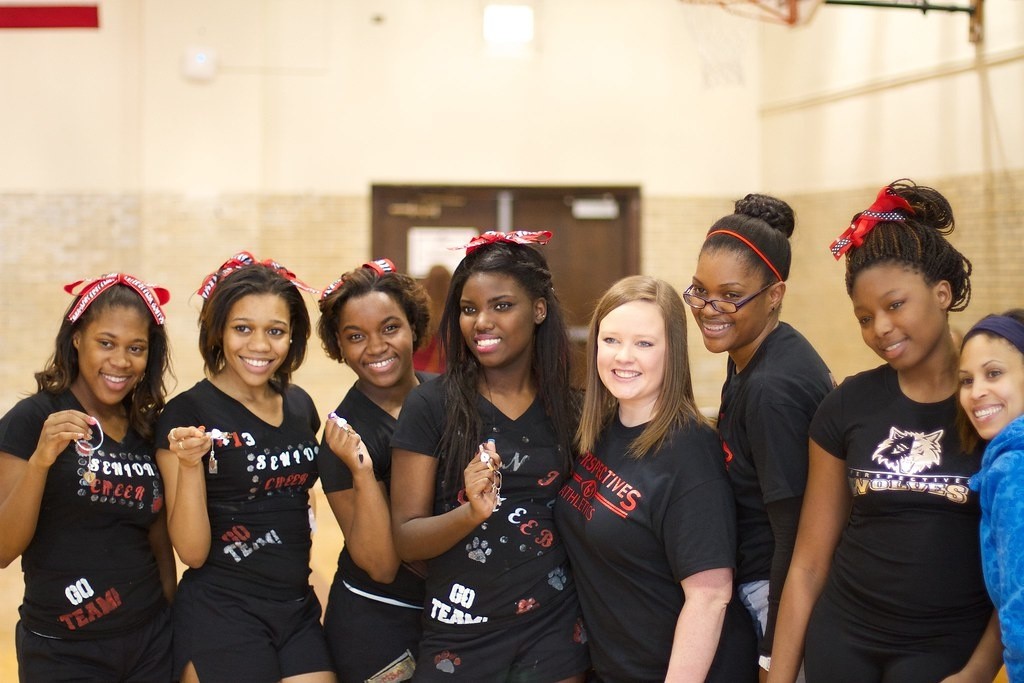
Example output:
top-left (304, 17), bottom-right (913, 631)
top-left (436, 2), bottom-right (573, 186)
top-left (758, 655), bottom-right (770, 671)
top-left (204, 430), bottom-right (234, 469)
top-left (328, 412), bottom-right (350, 433)
top-left (73, 416), bottom-right (103, 483)
top-left (479, 444), bottom-right (502, 504)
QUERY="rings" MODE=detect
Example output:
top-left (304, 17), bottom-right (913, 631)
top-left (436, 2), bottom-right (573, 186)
top-left (170, 428), bottom-right (177, 440)
top-left (179, 442), bottom-right (184, 450)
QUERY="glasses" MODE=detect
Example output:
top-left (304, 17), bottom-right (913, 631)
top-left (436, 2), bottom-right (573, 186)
top-left (682, 280), bottom-right (779, 313)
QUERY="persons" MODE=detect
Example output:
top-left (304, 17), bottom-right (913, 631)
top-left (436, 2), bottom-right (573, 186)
top-left (767, 178), bottom-right (1004, 683)
top-left (550, 276), bottom-right (759, 683)
top-left (0, 272), bottom-right (177, 683)
top-left (317, 257), bottom-right (443, 683)
top-left (954, 309), bottom-right (1024, 683)
top-left (388, 230), bottom-right (591, 683)
top-left (682, 193), bottom-right (837, 683)
top-left (152, 252), bottom-right (337, 683)
